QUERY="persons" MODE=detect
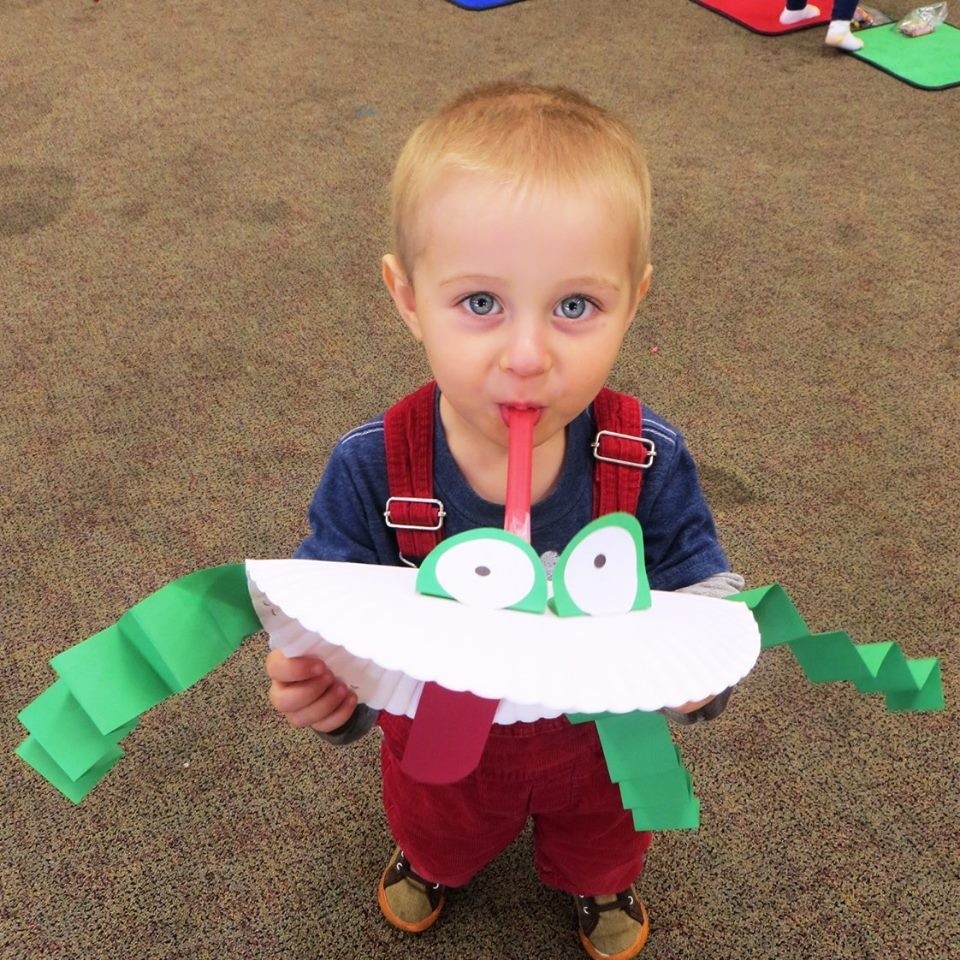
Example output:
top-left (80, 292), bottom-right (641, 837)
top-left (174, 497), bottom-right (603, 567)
top-left (264, 80), bottom-right (745, 960)
top-left (781, 0), bottom-right (864, 51)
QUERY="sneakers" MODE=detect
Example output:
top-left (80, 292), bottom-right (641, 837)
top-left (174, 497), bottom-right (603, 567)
top-left (376, 845), bottom-right (446, 933)
top-left (571, 878), bottom-right (648, 960)
